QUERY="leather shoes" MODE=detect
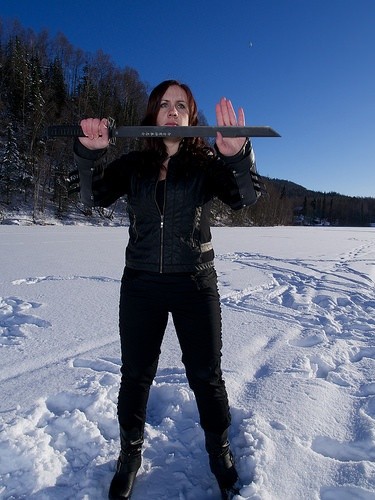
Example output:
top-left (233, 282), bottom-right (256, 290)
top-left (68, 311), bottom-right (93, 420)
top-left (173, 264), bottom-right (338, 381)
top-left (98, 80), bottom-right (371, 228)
top-left (108, 451), bottom-right (142, 500)
top-left (207, 441), bottom-right (243, 498)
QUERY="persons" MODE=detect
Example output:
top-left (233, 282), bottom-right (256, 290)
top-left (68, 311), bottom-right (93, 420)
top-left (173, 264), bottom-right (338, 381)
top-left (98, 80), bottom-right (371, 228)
top-left (72, 80), bottom-right (261, 500)
